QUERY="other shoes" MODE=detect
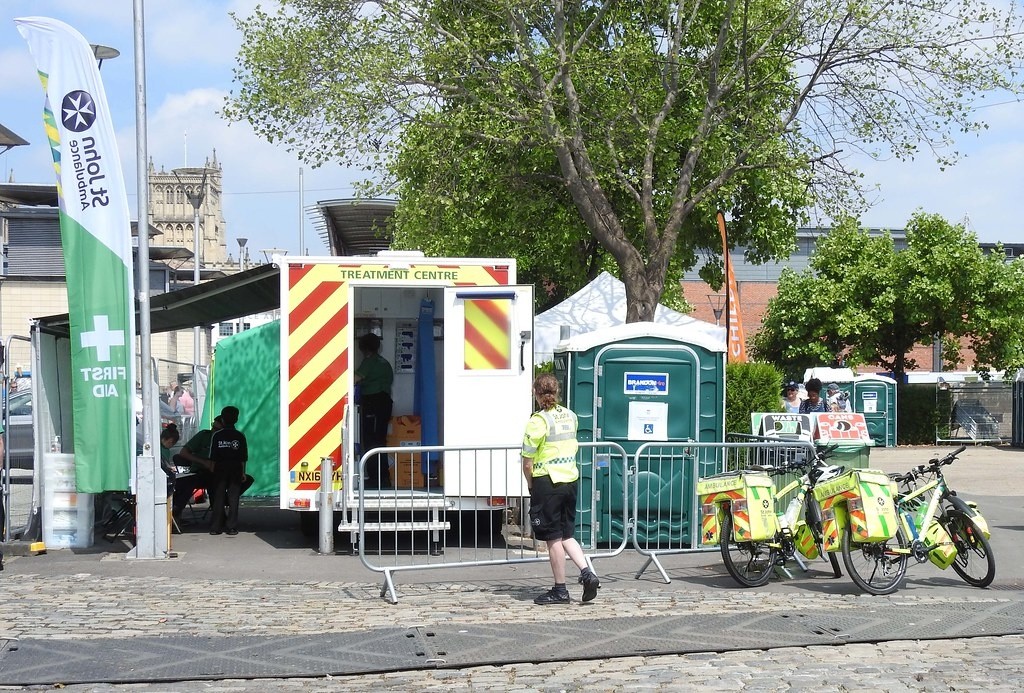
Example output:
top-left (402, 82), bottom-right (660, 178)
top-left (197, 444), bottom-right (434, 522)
top-left (227, 529), bottom-right (238, 535)
top-left (210, 529), bottom-right (222, 535)
top-left (363, 479), bottom-right (391, 488)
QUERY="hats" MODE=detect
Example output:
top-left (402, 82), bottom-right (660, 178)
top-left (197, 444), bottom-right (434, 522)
top-left (827, 384), bottom-right (838, 390)
top-left (787, 382), bottom-right (799, 389)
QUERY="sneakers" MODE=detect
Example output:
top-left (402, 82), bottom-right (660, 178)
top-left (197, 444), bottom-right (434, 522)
top-left (534, 587), bottom-right (570, 604)
top-left (582, 572), bottom-right (599, 602)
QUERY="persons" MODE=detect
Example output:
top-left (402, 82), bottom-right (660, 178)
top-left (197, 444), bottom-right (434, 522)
top-left (168, 381), bottom-right (195, 415)
top-left (180, 415), bottom-right (254, 506)
top-left (353, 333), bottom-right (393, 489)
top-left (136, 423), bottom-right (180, 466)
top-left (521, 374), bottom-right (599, 604)
top-left (781, 384), bottom-right (803, 414)
top-left (209, 406), bottom-right (248, 535)
top-left (0, 423), bottom-right (6, 572)
top-left (827, 383), bottom-right (852, 412)
top-left (799, 378), bottom-right (833, 414)
top-left (170, 385), bottom-right (185, 415)
top-left (9, 382), bottom-right (17, 393)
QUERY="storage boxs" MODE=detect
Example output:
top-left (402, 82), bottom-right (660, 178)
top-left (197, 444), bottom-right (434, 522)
top-left (43, 452), bottom-right (95, 549)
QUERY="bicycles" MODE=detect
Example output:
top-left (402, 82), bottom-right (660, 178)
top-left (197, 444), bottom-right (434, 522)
top-left (713, 439), bottom-right (842, 587)
top-left (837, 445), bottom-right (996, 596)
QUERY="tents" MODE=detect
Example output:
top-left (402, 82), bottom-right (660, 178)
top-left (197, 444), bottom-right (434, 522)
top-left (532, 269), bottom-right (726, 364)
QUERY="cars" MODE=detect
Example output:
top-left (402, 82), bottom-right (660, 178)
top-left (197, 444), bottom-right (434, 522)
top-left (1, 388), bottom-right (185, 460)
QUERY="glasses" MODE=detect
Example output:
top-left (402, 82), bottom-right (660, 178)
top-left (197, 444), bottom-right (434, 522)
top-left (211, 423), bottom-right (221, 431)
top-left (788, 389), bottom-right (796, 391)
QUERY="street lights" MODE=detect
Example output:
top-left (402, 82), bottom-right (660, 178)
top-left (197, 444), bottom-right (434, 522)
top-left (234, 238), bottom-right (250, 333)
top-left (706, 293), bottom-right (728, 327)
top-left (171, 165), bottom-right (221, 373)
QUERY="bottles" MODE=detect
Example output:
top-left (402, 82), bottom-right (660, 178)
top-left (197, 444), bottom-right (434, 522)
top-left (777, 510), bottom-right (788, 527)
top-left (904, 511), bottom-right (919, 539)
top-left (915, 501), bottom-right (928, 529)
top-left (785, 497), bottom-right (799, 523)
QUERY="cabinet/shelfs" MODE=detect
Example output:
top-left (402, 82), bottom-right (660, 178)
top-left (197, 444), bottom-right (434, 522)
top-left (428, 288), bottom-right (444, 320)
top-left (355, 288), bottom-right (428, 319)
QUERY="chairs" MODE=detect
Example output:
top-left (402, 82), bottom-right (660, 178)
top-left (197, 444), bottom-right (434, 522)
top-left (172, 454), bottom-right (211, 525)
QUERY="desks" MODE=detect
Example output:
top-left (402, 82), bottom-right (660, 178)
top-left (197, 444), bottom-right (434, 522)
top-left (172, 472), bottom-right (200, 533)
